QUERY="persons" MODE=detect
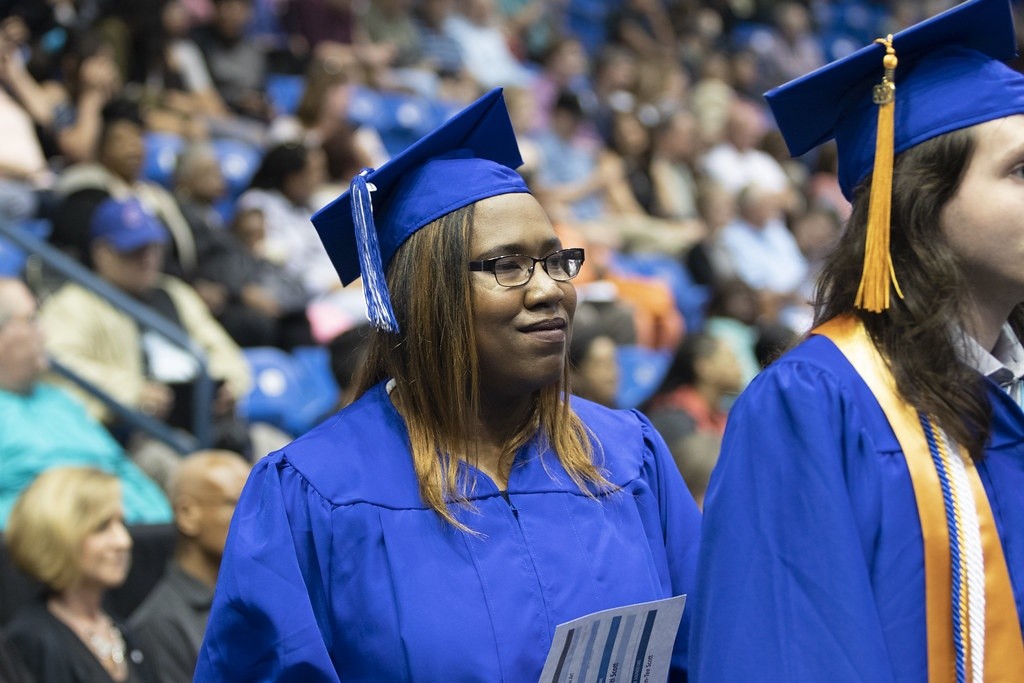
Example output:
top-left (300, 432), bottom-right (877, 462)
top-left (689, 0), bottom-right (1024, 683)
top-left (1, 0), bottom-right (954, 530)
top-left (0, 465), bottom-right (161, 682)
top-left (187, 84), bottom-right (706, 683)
top-left (119, 447), bottom-right (254, 683)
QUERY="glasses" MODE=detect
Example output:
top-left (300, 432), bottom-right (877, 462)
top-left (469, 249), bottom-right (585, 286)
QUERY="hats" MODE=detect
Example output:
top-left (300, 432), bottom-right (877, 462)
top-left (762, 0), bottom-right (1024, 311)
top-left (311, 87), bottom-right (531, 334)
top-left (92, 198), bottom-right (167, 251)
